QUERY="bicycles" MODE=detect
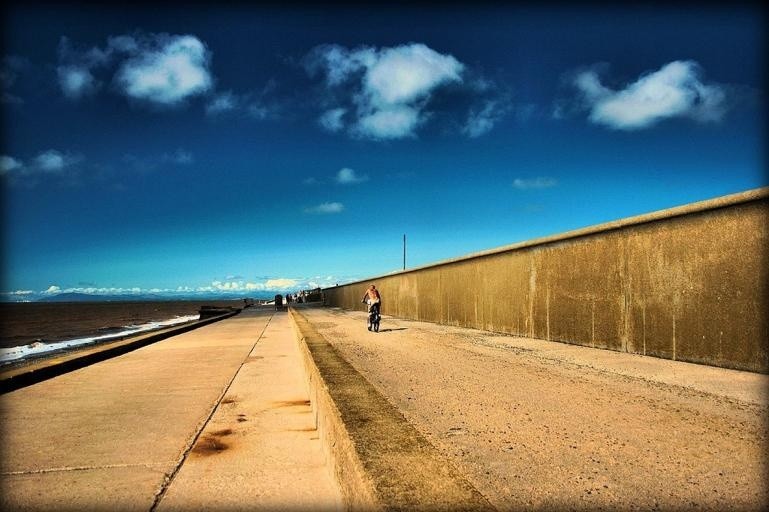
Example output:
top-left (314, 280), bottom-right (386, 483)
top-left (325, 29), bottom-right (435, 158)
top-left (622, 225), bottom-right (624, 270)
top-left (361, 298), bottom-right (383, 333)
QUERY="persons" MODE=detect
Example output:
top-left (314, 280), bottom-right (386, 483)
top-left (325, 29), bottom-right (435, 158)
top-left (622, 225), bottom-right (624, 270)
top-left (361, 285), bottom-right (381, 330)
top-left (286, 291), bottom-right (306, 304)
